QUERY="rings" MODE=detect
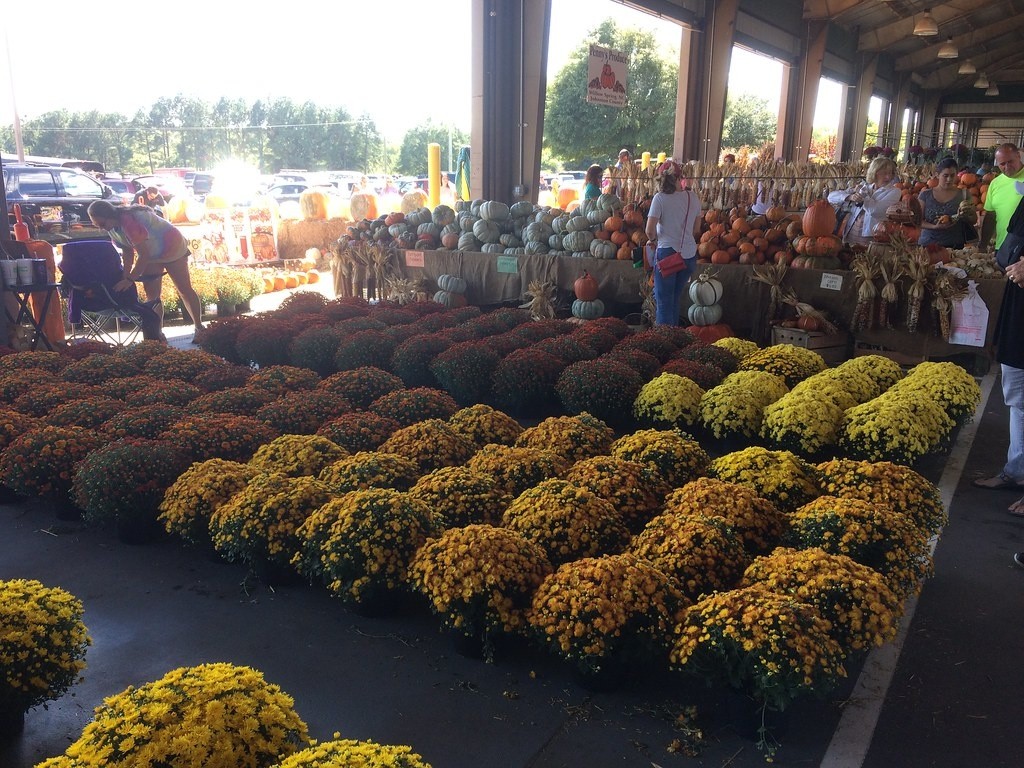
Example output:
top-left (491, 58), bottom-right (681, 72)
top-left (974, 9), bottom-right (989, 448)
top-left (1009, 274), bottom-right (1015, 280)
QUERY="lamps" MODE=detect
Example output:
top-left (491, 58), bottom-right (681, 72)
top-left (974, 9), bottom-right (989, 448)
top-left (913, 1), bottom-right (1000, 97)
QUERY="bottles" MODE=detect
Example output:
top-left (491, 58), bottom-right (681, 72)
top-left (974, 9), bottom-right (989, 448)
top-left (854, 182), bottom-right (868, 207)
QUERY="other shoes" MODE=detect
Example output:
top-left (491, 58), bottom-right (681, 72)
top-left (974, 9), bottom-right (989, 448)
top-left (191, 326), bottom-right (205, 344)
top-left (1014, 552), bottom-right (1024, 567)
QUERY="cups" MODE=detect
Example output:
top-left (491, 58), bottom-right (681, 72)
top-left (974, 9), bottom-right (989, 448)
top-left (16, 258), bottom-right (34, 286)
top-left (33, 258), bottom-right (47, 285)
top-left (0, 259), bottom-right (19, 285)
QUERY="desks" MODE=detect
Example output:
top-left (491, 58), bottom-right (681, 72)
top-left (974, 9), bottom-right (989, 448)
top-left (0, 282), bottom-right (63, 352)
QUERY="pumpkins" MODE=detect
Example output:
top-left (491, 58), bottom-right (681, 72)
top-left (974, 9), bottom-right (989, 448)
top-left (398, 188), bottom-right (430, 216)
top-left (258, 247), bottom-right (333, 292)
top-left (432, 273), bottom-right (467, 308)
top-left (299, 186), bottom-right (327, 220)
top-left (924, 237), bottom-right (1002, 278)
top-left (696, 198), bottom-right (848, 271)
top-left (892, 172), bottom-right (996, 223)
top-left (685, 278), bottom-right (734, 339)
top-left (873, 220), bottom-right (920, 244)
top-left (567, 275), bottom-right (604, 325)
top-left (337, 193), bottom-right (651, 261)
top-left (167, 193), bottom-right (224, 224)
top-left (798, 316), bottom-right (819, 331)
top-left (782, 320), bottom-right (797, 327)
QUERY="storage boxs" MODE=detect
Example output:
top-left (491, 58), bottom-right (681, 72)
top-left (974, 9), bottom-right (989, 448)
top-left (765, 324), bottom-right (848, 372)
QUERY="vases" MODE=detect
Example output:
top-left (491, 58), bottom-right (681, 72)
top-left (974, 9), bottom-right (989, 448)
top-left (179, 298), bottom-right (194, 323)
top-left (234, 299), bottom-right (250, 313)
top-left (216, 300), bottom-right (236, 317)
top-left (0, 682), bottom-right (25, 740)
top-left (0, 481), bottom-right (788, 740)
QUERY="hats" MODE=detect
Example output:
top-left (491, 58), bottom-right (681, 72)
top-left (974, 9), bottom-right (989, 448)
top-left (659, 161), bottom-right (680, 175)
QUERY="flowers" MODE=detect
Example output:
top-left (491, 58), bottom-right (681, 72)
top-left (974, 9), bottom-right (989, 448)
top-left (0, 293), bottom-right (983, 709)
top-left (0, 577), bottom-right (97, 713)
top-left (134, 267), bottom-right (261, 308)
top-left (29, 661), bottom-right (310, 768)
top-left (269, 732), bottom-right (432, 768)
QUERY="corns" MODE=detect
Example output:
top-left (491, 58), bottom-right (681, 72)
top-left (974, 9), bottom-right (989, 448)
top-left (818, 317), bottom-right (839, 337)
top-left (848, 297), bottom-right (950, 342)
top-left (609, 185), bottom-right (659, 204)
top-left (340, 275), bottom-right (389, 301)
top-left (686, 188), bottom-right (833, 209)
top-left (767, 304), bottom-right (783, 325)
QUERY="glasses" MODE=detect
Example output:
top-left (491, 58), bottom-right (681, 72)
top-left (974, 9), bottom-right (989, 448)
top-left (596, 176), bottom-right (602, 179)
top-left (95, 215), bottom-right (110, 227)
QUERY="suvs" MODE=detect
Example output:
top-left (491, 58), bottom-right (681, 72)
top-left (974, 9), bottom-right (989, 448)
top-left (1, 160), bottom-right (129, 235)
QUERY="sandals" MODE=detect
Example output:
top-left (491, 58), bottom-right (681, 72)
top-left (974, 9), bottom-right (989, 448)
top-left (973, 472), bottom-right (1024, 488)
top-left (1010, 497), bottom-right (1024, 515)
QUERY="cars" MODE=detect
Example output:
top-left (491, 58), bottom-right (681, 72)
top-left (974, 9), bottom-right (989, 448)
top-left (0, 156), bottom-right (429, 224)
top-left (540, 171), bottom-right (586, 189)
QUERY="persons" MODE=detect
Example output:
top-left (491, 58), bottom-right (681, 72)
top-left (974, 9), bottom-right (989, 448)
top-left (724, 154), bottom-right (735, 167)
top-left (827, 157), bottom-right (902, 248)
top-left (585, 166), bottom-right (604, 201)
top-left (747, 176), bottom-right (774, 217)
top-left (134, 187), bottom-right (166, 216)
top-left (645, 160), bottom-right (702, 326)
top-left (978, 143), bottom-right (1024, 566)
top-left (87, 200), bottom-right (207, 346)
top-left (615, 148), bottom-right (632, 174)
top-left (917, 158), bottom-right (982, 254)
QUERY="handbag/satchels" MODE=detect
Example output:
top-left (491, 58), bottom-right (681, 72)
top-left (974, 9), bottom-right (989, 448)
top-left (656, 252), bottom-right (688, 278)
top-left (962, 188), bottom-right (979, 242)
top-left (833, 201), bottom-right (850, 234)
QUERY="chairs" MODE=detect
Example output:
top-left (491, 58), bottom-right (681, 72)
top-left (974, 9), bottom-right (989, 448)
top-left (61, 241), bottom-right (162, 346)
